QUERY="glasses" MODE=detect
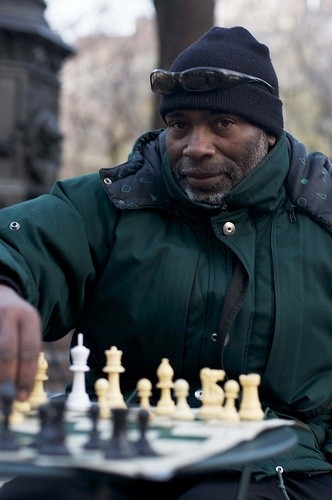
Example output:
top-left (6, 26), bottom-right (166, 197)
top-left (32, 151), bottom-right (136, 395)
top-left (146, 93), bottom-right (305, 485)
top-left (150, 67), bottom-right (278, 98)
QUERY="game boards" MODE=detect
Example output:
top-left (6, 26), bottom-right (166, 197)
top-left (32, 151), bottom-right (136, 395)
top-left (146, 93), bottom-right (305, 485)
top-left (1, 390), bottom-right (300, 481)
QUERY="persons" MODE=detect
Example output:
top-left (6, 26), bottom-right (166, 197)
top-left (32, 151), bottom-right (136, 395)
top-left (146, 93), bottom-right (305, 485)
top-left (0, 26), bottom-right (332, 500)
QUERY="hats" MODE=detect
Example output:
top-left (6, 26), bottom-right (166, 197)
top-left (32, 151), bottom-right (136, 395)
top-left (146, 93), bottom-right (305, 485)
top-left (158, 26), bottom-right (284, 140)
